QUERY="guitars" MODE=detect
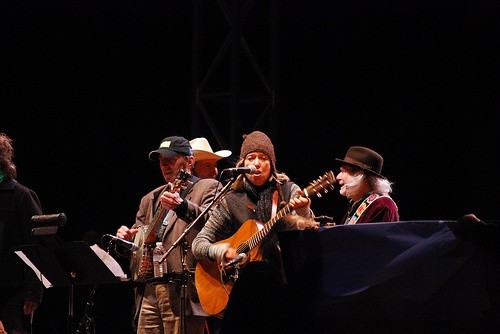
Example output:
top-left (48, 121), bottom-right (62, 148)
top-left (195, 170), bottom-right (337, 320)
top-left (129, 169), bottom-right (194, 284)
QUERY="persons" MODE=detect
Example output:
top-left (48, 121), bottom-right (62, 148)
top-left (115, 136), bottom-right (224, 334)
top-left (0, 133), bottom-right (44, 334)
top-left (192, 131), bottom-right (320, 334)
top-left (323, 147), bottom-right (400, 225)
top-left (189, 137), bottom-right (232, 179)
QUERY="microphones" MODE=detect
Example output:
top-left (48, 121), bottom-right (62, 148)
top-left (225, 253), bottom-right (247, 267)
top-left (223, 165), bottom-right (257, 175)
top-left (104, 234), bottom-right (140, 251)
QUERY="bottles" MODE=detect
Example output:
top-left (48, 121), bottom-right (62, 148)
top-left (153, 242), bottom-right (168, 278)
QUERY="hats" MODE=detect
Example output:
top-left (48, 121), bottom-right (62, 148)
top-left (336, 146), bottom-right (386, 179)
top-left (240, 131), bottom-right (275, 164)
top-left (149, 135), bottom-right (192, 159)
top-left (188, 137), bottom-right (232, 161)
